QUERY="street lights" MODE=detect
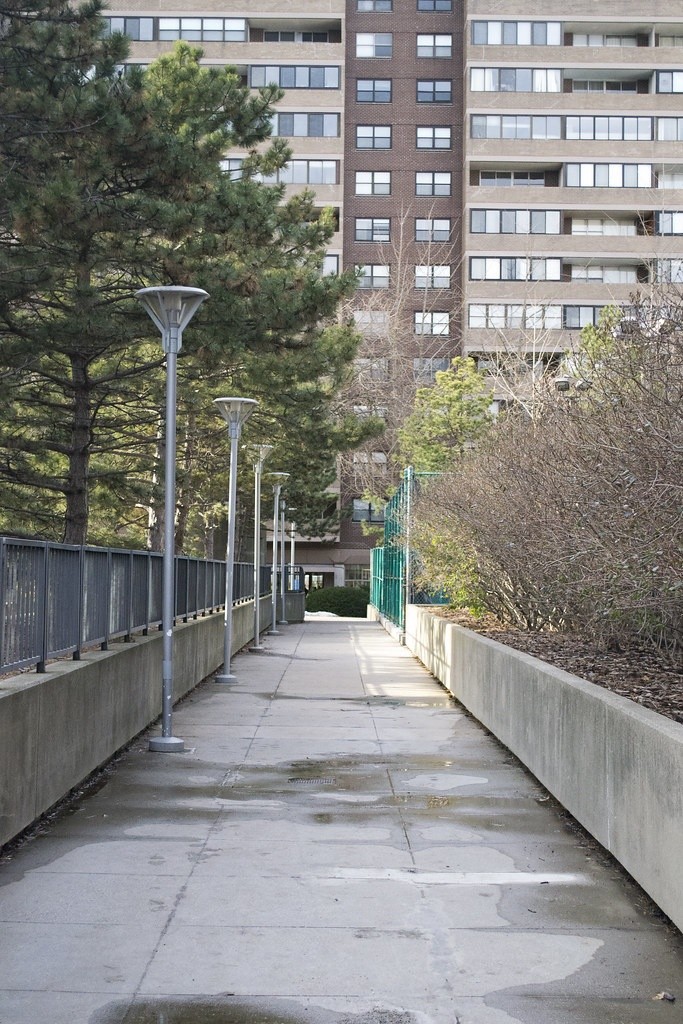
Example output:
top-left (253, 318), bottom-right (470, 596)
top-left (278, 495), bottom-right (292, 626)
top-left (264, 472), bottom-right (291, 636)
top-left (289, 507), bottom-right (298, 592)
top-left (240, 443), bottom-right (274, 655)
top-left (131, 284), bottom-right (209, 752)
top-left (208, 396), bottom-right (261, 685)
